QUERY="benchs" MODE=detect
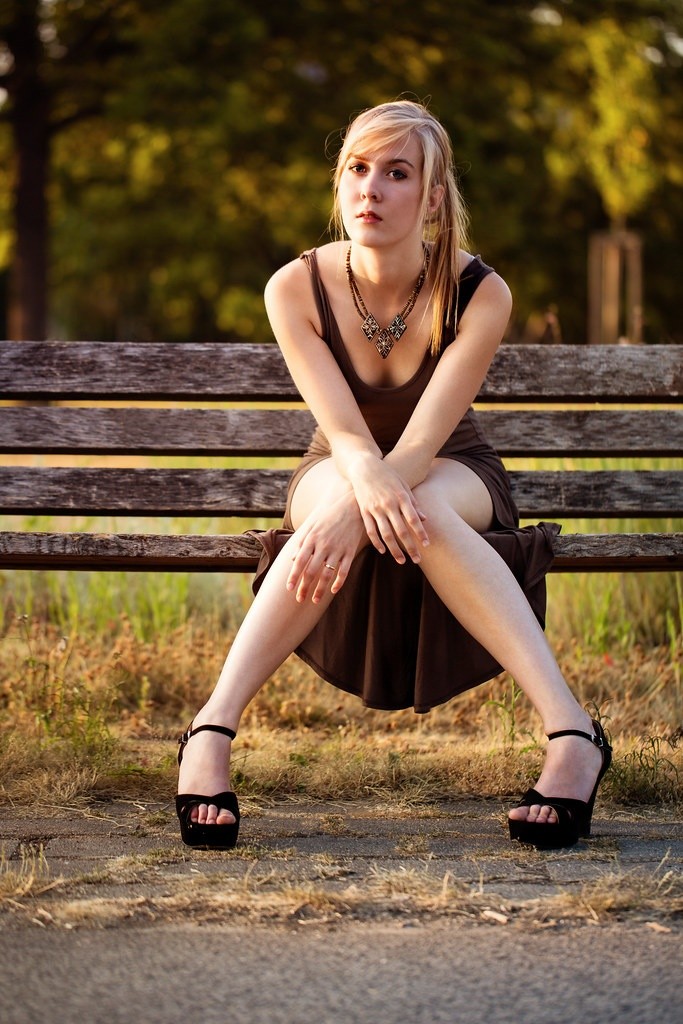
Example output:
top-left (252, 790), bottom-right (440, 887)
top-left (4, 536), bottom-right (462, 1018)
top-left (0, 339), bottom-right (682, 574)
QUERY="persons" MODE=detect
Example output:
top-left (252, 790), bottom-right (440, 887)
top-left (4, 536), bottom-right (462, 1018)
top-left (176, 101), bottom-right (612, 850)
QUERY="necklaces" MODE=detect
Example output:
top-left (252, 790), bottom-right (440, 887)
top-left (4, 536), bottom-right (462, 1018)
top-left (345, 240), bottom-right (430, 359)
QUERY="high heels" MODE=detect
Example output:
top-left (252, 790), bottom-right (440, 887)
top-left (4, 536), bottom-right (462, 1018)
top-left (174, 718), bottom-right (241, 850)
top-left (508, 719), bottom-right (613, 849)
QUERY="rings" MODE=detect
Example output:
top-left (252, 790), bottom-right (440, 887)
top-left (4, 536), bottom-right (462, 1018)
top-left (325, 564), bottom-right (335, 570)
